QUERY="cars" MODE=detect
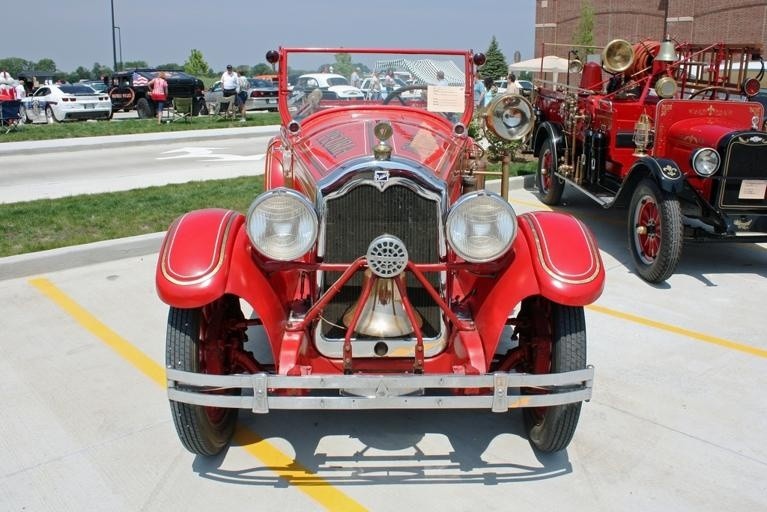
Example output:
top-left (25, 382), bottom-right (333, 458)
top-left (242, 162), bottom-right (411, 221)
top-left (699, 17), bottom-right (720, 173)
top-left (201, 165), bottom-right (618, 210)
top-left (533, 33), bottom-right (767, 282)
top-left (19, 83), bottom-right (113, 123)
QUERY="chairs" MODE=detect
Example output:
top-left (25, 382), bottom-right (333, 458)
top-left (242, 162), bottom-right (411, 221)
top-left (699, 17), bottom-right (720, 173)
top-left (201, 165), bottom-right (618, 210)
top-left (167, 96), bottom-right (193, 124)
top-left (0, 100), bottom-right (23, 134)
top-left (208, 100), bottom-right (231, 123)
top-left (595, 97), bottom-right (656, 147)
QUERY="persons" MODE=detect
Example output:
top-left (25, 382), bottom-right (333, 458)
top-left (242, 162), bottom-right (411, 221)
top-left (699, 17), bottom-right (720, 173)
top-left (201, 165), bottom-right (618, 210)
top-left (384, 68), bottom-right (396, 94)
top-left (473, 73), bottom-right (486, 113)
top-left (435, 71), bottom-right (449, 87)
top-left (236, 70), bottom-right (251, 122)
top-left (0, 68), bottom-right (25, 134)
top-left (369, 70), bottom-right (382, 101)
top-left (349, 67), bottom-right (363, 89)
top-left (320, 65), bottom-right (329, 74)
top-left (146, 71), bottom-right (169, 124)
top-left (220, 64), bottom-right (238, 98)
top-left (504, 73), bottom-right (520, 96)
top-left (484, 75), bottom-right (498, 106)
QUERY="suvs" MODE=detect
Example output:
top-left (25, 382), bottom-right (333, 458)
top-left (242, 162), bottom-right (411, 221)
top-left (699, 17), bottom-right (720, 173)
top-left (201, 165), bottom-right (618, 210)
top-left (108, 67), bottom-right (207, 117)
